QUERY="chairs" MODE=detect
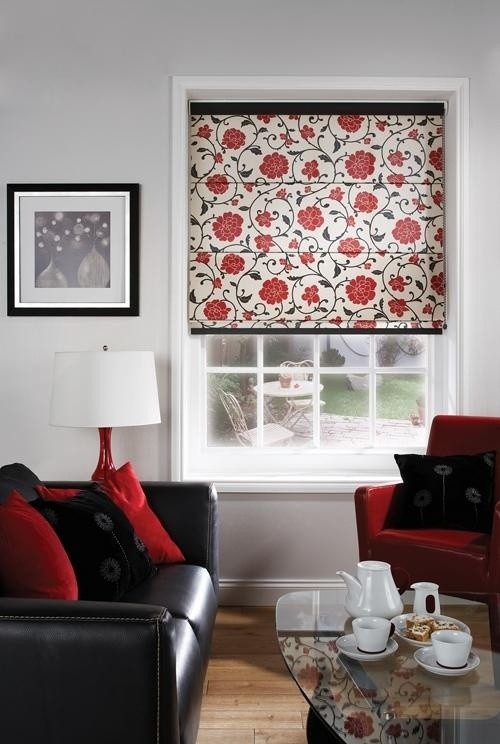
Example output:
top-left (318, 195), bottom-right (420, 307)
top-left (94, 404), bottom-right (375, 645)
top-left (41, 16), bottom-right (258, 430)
top-left (281, 360), bottom-right (326, 437)
top-left (215, 387), bottom-right (295, 449)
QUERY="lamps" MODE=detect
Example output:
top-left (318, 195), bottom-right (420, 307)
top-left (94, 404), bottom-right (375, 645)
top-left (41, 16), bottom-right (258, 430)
top-left (47, 345), bottom-right (165, 480)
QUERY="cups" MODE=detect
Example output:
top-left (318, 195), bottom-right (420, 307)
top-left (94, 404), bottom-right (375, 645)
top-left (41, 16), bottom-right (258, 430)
top-left (431, 630), bottom-right (473, 667)
top-left (352, 615), bottom-right (391, 651)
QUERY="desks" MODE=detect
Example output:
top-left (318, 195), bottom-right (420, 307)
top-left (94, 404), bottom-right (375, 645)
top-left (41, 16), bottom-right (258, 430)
top-left (254, 381), bottom-right (325, 444)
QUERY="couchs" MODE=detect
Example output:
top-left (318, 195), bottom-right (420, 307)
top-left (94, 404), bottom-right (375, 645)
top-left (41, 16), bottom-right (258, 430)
top-left (0, 459), bottom-right (223, 743)
top-left (352, 411), bottom-right (500, 687)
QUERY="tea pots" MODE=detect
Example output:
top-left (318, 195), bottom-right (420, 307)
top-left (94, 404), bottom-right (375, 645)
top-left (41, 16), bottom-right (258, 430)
top-left (410, 579), bottom-right (441, 616)
top-left (334, 558), bottom-right (404, 618)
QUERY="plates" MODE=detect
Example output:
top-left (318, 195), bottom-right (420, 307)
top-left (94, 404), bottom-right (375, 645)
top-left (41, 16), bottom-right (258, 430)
top-left (415, 646), bottom-right (480, 675)
top-left (334, 634), bottom-right (399, 660)
top-left (389, 612), bottom-right (472, 646)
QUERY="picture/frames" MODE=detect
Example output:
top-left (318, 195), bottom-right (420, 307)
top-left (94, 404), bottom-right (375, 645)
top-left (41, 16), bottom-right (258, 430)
top-left (3, 179), bottom-right (142, 319)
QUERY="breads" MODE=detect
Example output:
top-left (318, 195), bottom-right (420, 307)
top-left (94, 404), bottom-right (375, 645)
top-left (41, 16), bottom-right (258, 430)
top-left (406, 615), bottom-right (461, 642)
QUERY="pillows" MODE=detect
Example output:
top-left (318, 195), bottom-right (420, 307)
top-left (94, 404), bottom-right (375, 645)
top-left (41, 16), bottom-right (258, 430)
top-left (387, 443), bottom-right (498, 535)
top-left (0, 461), bottom-right (186, 602)
